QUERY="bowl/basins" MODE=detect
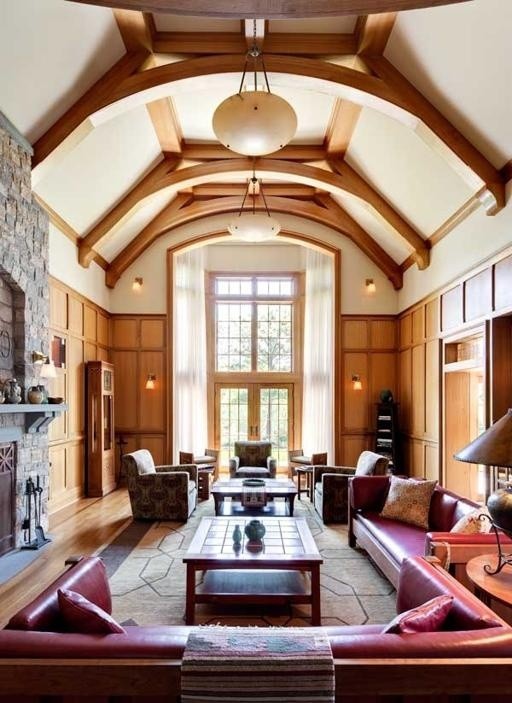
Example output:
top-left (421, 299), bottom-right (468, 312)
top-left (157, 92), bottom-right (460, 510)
top-left (47, 396), bottom-right (63, 404)
top-left (378, 437), bottom-right (392, 447)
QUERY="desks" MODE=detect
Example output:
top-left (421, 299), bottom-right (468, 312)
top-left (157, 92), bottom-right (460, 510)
top-left (466, 553), bottom-right (512, 608)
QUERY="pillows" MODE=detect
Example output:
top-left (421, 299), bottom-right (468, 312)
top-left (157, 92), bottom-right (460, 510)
top-left (382, 595), bottom-right (454, 633)
top-left (58, 588), bottom-right (126, 633)
top-left (379, 476), bottom-right (438, 529)
top-left (450, 506), bottom-right (493, 534)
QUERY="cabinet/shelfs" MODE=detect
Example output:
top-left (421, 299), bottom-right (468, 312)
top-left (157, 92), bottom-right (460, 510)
top-left (373, 402), bottom-right (403, 474)
top-left (86, 360), bottom-right (118, 497)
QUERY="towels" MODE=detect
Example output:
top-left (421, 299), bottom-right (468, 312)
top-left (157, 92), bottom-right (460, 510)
top-left (180, 624), bottom-right (335, 703)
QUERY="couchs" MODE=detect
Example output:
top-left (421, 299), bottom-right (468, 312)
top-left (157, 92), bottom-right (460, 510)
top-left (346, 474), bottom-right (512, 594)
top-left (0, 556), bottom-right (512, 703)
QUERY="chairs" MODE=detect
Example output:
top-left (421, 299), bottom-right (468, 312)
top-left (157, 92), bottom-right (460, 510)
top-left (122, 442), bottom-right (389, 522)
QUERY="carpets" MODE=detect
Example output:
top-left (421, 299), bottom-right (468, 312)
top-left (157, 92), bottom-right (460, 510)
top-left (97, 491), bottom-right (399, 626)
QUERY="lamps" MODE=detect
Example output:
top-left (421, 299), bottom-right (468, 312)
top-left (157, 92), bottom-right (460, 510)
top-left (134, 278), bottom-right (143, 290)
top-left (146, 375), bottom-right (155, 390)
top-left (228, 171), bottom-right (281, 242)
top-left (352, 375), bottom-right (363, 389)
top-left (365, 279), bottom-right (376, 294)
top-left (453, 409), bottom-right (512, 574)
top-left (33, 350), bottom-right (57, 378)
top-left (213, 19), bottom-right (297, 155)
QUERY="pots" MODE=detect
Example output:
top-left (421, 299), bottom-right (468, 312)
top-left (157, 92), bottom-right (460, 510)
top-left (378, 452), bottom-right (393, 465)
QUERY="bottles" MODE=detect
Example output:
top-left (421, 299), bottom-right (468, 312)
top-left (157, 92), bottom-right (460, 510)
top-left (27, 386), bottom-right (44, 403)
top-left (8, 382), bottom-right (22, 403)
top-left (231, 524), bottom-right (242, 543)
top-left (245, 519), bottom-right (265, 541)
top-left (37, 384), bottom-right (49, 405)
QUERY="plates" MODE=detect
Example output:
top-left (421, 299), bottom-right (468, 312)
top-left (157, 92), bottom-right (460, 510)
top-left (378, 415), bottom-right (392, 420)
top-left (378, 428), bottom-right (390, 432)
top-left (243, 478), bottom-right (265, 485)
